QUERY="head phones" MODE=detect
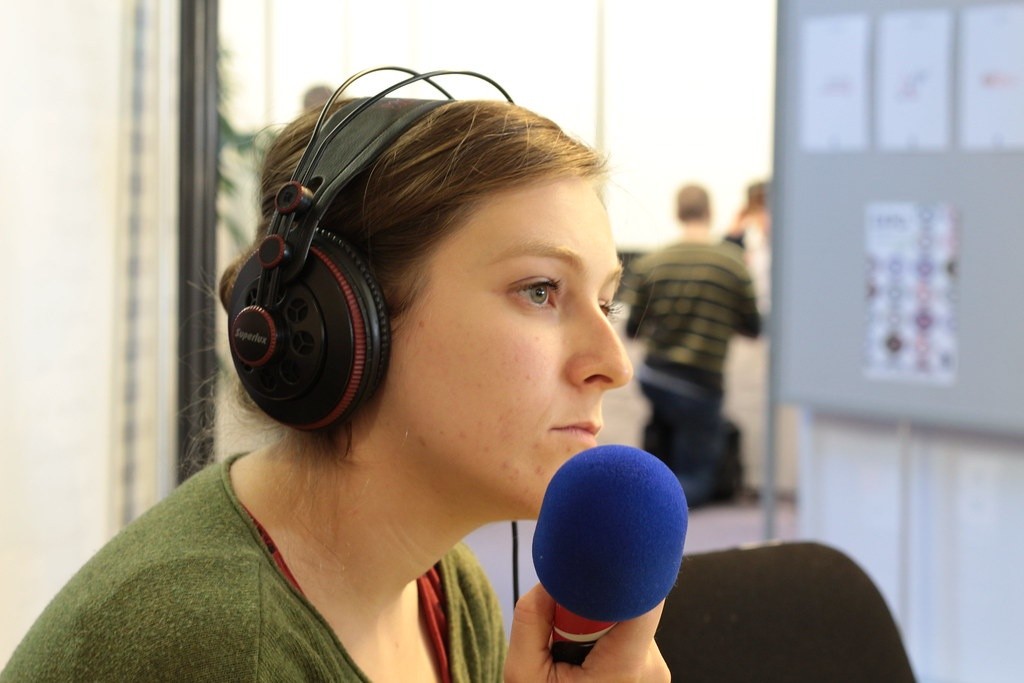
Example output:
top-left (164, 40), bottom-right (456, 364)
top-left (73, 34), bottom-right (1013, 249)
top-left (226, 68), bottom-right (516, 434)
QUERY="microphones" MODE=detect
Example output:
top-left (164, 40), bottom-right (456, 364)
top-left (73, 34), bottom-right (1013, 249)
top-left (526, 446), bottom-right (687, 667)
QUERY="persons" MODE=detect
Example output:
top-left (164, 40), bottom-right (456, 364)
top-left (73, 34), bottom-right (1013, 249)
top-left (724, 181), bottom-right (773, 316)
top-left (2, 96), bottom-right (673, 683)
top-left (624, 183), bottom-right (763, 510)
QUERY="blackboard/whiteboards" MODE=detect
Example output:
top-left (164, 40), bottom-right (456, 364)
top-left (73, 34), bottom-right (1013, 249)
top-left (764, 0), bottom-right (1024, 440)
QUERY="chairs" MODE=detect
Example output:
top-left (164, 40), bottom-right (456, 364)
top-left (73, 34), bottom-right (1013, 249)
top-left (654, 542), bottom-right (915, 682)
